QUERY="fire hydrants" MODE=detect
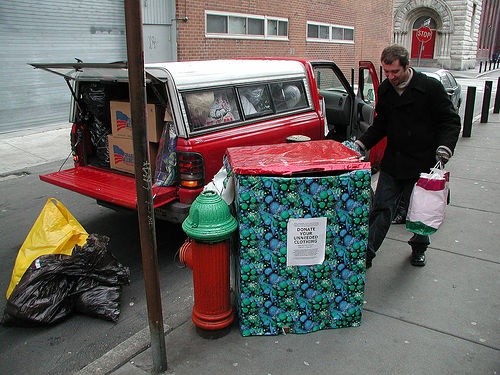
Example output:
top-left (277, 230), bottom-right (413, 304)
top-left (174, 188), bottom-right (239, 339)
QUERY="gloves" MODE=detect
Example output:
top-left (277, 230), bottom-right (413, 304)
top-left (437, 147), bottom-right (451, 165)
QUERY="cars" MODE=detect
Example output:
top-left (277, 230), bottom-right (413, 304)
top-left (322, 66), bottom-right (462, 118)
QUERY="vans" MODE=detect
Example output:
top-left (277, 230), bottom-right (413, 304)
top-left (26, 57), bottom-right (387, 225)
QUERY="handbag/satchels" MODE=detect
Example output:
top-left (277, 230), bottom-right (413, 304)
top-left (6, 198), bottom-right (90, 299)
top-left (406, 161), bottom-right (450, 235)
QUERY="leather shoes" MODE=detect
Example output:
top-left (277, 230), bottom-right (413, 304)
top-left (411, 249), bottom-right (426, 266)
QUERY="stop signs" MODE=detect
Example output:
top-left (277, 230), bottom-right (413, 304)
top-left (416, 26), bottom-right (432, 43)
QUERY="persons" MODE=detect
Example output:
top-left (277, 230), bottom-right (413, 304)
top-left (354, 45), bottom-right (462, 269)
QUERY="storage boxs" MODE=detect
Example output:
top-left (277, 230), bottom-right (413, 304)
top-left (107, 100), bottom-right (161, 177)
top-left (225, 139), bottom-right (373, 337)
